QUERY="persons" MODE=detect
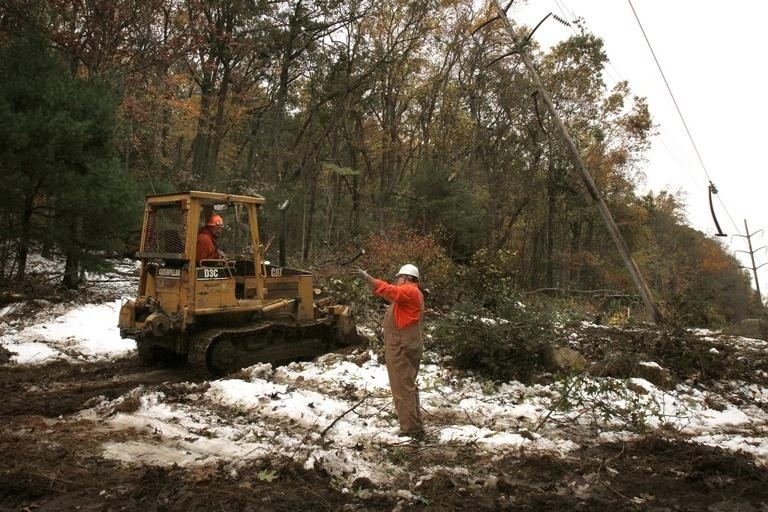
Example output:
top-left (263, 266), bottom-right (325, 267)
top-left (194, 213), bottom-right (226, 267)
top-left (348, 263), bottom-right (426, 437)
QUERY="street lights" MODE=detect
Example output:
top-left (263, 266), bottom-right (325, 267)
top-left (276, 197), bottom-right (293, 264)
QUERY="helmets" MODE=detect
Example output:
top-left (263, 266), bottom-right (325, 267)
top-left (394, 264), bottom-right (420, 281)
top-left (206, 214), bottom-right (225, 227)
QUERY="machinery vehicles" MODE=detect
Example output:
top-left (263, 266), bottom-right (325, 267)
top-left (117, 189), bottom-right (368, 377)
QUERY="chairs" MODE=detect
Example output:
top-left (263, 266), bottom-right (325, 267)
top-left (164, 229), bottom-right (189, 270)
